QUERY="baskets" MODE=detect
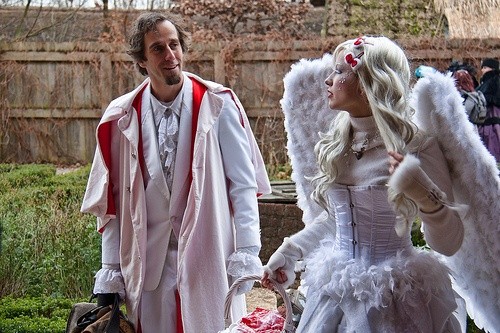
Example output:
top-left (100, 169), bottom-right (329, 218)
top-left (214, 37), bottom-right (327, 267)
top-left (218, 275), bottom-right (295, 333)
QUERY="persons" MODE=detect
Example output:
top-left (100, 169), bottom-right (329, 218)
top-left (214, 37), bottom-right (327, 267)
top-left (476, 57), bottom-right (500, 170)
top-left (261, 35), bottom-right (467, 333)
top-left (454, 69), bottom-right (486, 136)
top-left (80, 12), bottom-right (272, 333)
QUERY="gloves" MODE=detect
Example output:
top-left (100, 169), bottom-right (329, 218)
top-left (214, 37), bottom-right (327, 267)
top-left (229, 276), bottom-right (257, 296)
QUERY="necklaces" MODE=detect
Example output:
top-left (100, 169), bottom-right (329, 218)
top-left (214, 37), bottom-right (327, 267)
top-left (347, 127), bottom-right (379, 160)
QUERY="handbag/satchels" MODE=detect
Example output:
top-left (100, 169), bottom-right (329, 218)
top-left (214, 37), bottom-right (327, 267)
top-left (64, 293), bottom-right (135, 333)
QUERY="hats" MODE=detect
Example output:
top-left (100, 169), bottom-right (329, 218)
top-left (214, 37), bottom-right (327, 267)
top-left (481, 56), bottom-right (500, 72)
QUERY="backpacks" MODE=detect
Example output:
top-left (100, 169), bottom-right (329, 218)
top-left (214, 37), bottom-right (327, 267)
top-left (462, 90), bottom-right (487, 119)
